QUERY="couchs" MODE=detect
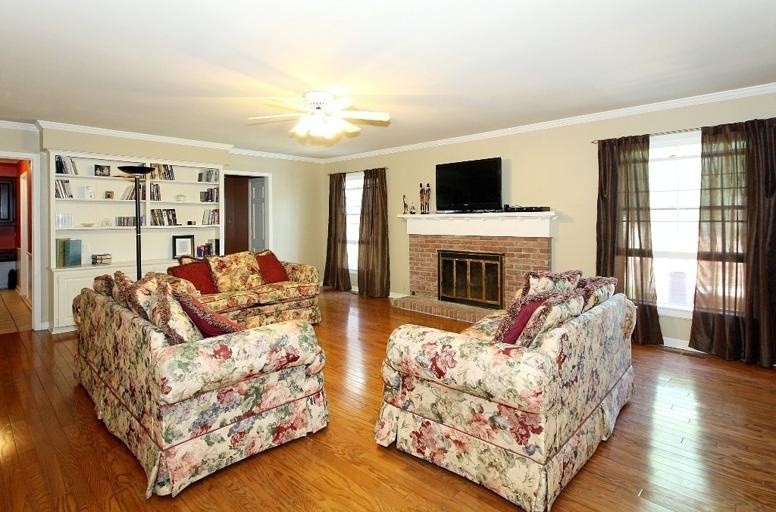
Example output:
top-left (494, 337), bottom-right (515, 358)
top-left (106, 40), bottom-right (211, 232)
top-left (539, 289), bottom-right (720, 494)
top-left (72, 288), bottom-right (329, 499)
top-left (370, 269), bottom-right (637, 512)
top-left (167, 250), bottom-right (322, 325)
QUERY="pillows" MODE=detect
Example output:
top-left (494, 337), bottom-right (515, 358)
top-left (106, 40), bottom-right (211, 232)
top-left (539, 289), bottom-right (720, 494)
top-left (582, 277), bottom-right (618, 313)
top-left (204, 250), bottom-right (266, 292)
top-left (92, 271), bottom-right (246, 345)
top-left (167, 262), bottom-right (219, 293)
top-left (493, 295), bottom-right (547, 344)
top-left (521, 269), bottom-right (583, 296)
top-left (254, 250), bottom-right (289, 284)
top-left (514, 293), bottom-right (585, 350)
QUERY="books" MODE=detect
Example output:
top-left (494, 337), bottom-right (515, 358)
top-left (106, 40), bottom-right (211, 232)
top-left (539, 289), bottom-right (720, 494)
top-left (53, 154), bottom-right (219, 267)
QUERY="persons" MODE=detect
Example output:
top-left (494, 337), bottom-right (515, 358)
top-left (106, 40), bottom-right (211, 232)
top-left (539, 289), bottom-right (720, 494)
top-left (419, 183), bottom-right (425, 212)
top-left (424, 183), bottom-right (430, 212)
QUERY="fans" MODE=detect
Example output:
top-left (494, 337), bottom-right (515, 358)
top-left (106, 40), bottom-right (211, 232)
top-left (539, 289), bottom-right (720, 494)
top-left (248, 91), bottom-right (390, 138)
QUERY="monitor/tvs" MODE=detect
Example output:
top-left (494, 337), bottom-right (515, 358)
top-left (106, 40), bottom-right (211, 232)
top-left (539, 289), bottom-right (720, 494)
top-left (434, 156), bottom-right (503, 212)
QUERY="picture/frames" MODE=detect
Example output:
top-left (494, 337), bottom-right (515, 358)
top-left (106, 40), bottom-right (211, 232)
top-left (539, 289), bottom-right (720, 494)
top-left (172, 235), bottom-right (195, 259)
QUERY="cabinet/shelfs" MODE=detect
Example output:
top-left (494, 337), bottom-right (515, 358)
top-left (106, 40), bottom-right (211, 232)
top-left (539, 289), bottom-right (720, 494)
top-left (46, 148), bottom-right (225, 334)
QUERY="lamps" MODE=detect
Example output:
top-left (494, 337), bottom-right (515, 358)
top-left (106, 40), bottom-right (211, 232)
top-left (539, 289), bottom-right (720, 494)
top-left (116, 166), bottom-right (155, 282)
